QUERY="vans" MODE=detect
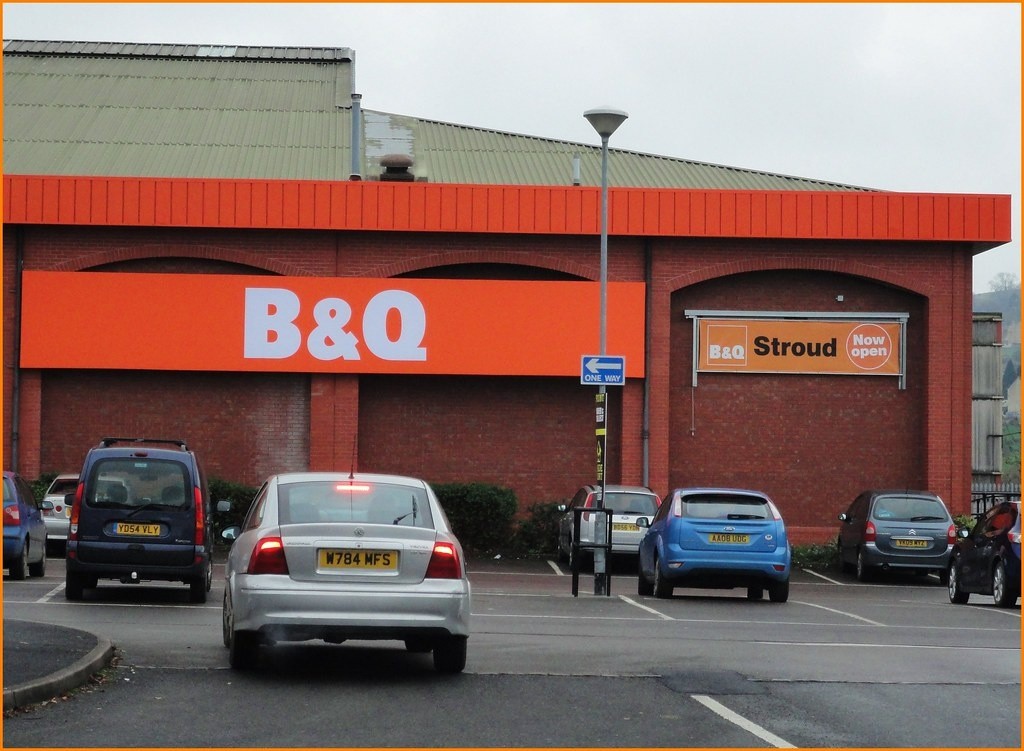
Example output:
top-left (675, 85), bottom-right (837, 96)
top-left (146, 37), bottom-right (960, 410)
top-left (64, 436), bottom-right (230, 603)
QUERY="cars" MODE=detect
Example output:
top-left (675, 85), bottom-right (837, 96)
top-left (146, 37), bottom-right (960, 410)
top-left (221, 472), bottom-right (470, 674)
top-left (837, 489), bottom-right (957, 584)
top-left (2, 470), bottom-right (54, 580)
top-left (556, 484), bottom-right (663, 572)
top-left (635, 486), bottom-right (790, 602)
top-left (41, 473), bottom-right (151, 558)
top-left (946, 500), bottom-right (1022, 608)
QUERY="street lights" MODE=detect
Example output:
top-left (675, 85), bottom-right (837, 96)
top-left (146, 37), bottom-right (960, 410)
top-left (583, 105), bottom-right (628, 597)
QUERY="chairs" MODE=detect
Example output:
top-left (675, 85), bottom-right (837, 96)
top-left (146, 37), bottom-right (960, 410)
top-left (107, 484), bottom-right (128, 504)
top-left (161, 485), bottom-right (185, 506)
